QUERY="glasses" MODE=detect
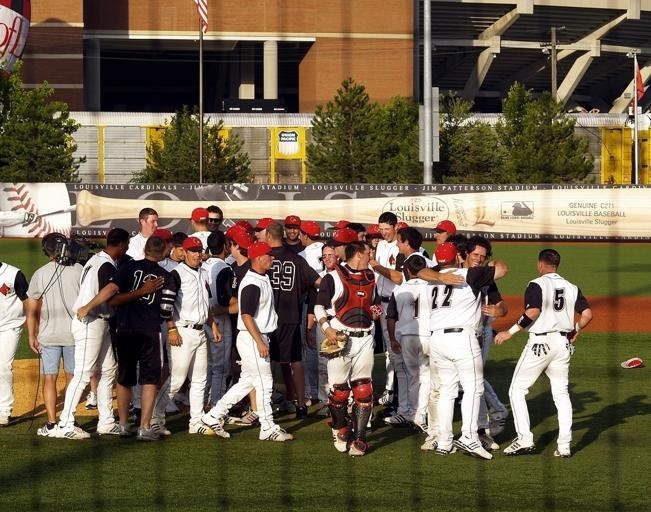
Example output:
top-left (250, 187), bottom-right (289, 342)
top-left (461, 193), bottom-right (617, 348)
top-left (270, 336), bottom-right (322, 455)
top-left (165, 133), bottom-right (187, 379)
top-left (435, 229), bottom-right (447, 234)
top-left (207, 217), bottom-right (221, 223)
top-left (285, 225), bottom-right (300, 229)
top-left (255, 228), bottom-right (265, 232)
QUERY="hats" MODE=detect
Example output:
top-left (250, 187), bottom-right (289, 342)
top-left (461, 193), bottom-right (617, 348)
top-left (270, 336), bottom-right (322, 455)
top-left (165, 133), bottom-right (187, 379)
top-left (189, 207), bottom-right (209, 224)
top-left (367, 225), bottom-right (381, 234)
top-left (247, 242), bottom-right (281, 260)
top-left (395, 222), bottom-right (408, 231)
top-left (430, 220), bottom-right (456, 234)
top-left (332, 229), bottom-right (358, 243)
top-left (434, 242), bottom-right (457, 263)
top-left (182, 237), bottom-right (205, 252)
top-left (300, 221), bottom-right (321, 236)
top-left (285, 215), bottom-right (301, 225)
top-left (252, 218), bottom-right (275, 231)
top-left (620, 358), bottom-right (644, 370)
top-left (328, 221), bottom-right (351, 230)
top-left (225, 221), bottom-right (254, 249)
top-left (154, 228), bottom-right (171, 241)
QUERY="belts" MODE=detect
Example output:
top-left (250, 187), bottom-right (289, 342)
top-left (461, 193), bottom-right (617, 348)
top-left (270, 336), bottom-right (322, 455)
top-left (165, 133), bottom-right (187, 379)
top-left (183, 324), bottom-right (207, 330)
top-left (344, 329), bottom-right (372, 338)
top-left (99, 315), bottom-right (108, 322)
top-left (429, 326), bottom-right (463, 334)
top-left (380, 296), bottom-right (391, 303)
top-left (536, 330), bottom-right (567, 338)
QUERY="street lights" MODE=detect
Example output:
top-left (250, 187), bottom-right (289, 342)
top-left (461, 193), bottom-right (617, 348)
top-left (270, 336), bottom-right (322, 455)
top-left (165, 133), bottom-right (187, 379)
top-left (626, 51), bottom-right (634, 61)
top-left (541, 47), bottom-right (557, 123)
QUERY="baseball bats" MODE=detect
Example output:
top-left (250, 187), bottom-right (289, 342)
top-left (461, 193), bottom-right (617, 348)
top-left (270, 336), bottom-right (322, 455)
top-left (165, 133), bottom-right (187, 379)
top-left (27, 205), bottom-right (76, 222)
top-left (77, 187), bottom-right (651, 237)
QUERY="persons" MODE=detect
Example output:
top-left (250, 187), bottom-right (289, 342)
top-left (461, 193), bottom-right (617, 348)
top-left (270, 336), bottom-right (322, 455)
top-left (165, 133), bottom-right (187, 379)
top-left (0, 260), bottom-right (37, 425)
top-left (493, 249), bottom-right (594, 459)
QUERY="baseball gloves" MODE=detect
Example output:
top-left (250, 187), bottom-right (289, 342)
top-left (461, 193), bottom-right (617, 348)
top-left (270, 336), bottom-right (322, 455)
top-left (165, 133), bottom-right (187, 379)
top-left (319, 331), bottom-right (348, 360)
top-left (621, 358), bottom-right (643, 369)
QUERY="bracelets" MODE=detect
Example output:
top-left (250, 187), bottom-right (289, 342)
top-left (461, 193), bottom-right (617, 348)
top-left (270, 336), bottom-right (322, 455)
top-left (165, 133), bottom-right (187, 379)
top-left (517, 313), bottom-right (533, 329)
top-left (508, 324), bottom-right (521, 337)
top-left (574, 322), bottom-right (582, 333)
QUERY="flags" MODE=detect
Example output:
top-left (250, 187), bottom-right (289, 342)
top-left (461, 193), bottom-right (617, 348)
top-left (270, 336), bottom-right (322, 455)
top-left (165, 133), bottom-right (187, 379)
top-left (195, 0), bottom-right (209, 34)
top-left (631, 64), bottom-right (645, 114)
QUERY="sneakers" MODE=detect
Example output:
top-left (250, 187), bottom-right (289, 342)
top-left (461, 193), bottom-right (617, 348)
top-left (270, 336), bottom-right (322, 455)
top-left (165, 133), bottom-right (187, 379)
top-left (503, 438), bottom-right (536, 454)
top-left (554, 448), bottom-right (571, 457)
top-left (320, 391), bottom-right (374, 457)
top-left (2, 416), bottom-right (9, 425)
top-left (37, 388), bottom-right (320, 442)
top-left (380, 393), bottom-right (507, 459)
